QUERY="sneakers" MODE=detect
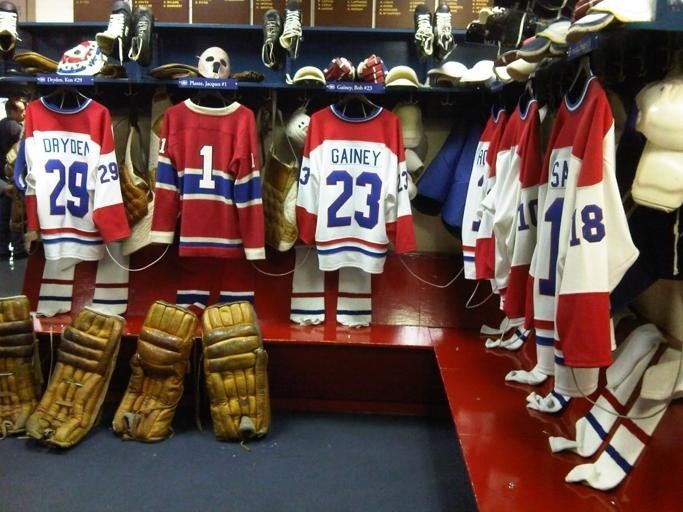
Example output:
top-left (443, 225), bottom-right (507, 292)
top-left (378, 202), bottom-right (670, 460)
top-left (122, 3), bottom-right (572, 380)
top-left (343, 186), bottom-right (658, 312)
top-left (95, 0), bottom-right (131, 67)
top-left (414, 4), bottom-right (435, 57)
top-left (433, 4), bottom-right (455, 60)
top-left (0, 1), bottom-right (23, 59)
top-left (127, 6), bottom-right (153, 67)
top-left (278, 0), bottom-right (304, 60)
top-left (261, 9), bottom-right (286, 71)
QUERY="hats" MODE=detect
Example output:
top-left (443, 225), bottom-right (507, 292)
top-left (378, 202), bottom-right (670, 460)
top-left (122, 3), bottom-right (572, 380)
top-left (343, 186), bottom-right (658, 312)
top-left (428, 62), bottom-right (468, 87)
top-left (459, 0), bottom-right (656, 84)
top-left (285, 66), bottom-right (327, 86)
top-left (384, 61), bottom-right (420, 89)
top-left (394, 103), bottom-right (425, 148)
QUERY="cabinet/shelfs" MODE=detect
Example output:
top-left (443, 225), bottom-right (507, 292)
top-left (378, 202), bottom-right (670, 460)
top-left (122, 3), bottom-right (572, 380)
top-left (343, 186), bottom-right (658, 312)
top-left (0, 22), bottom-right (682, 512)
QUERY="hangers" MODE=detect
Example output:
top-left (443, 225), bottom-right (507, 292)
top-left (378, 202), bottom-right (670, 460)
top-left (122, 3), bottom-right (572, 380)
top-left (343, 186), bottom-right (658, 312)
top-left (42, 86), bottom-right (89, 107)
top-left (189, 91), bottom-right (233, 108)
top-left (336, 95), bottom-right (378, 114)
top-left (519, 79), bottom-right (536, 101)
top-left (567, 54), bottom-right (595, 93)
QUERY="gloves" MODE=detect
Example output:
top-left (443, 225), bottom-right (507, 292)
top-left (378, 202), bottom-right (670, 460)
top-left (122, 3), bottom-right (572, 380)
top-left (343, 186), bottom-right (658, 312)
top-left (357, 54), bottom-right (389, 84)
top-left (323, 57), bottom-right (356, 82)
